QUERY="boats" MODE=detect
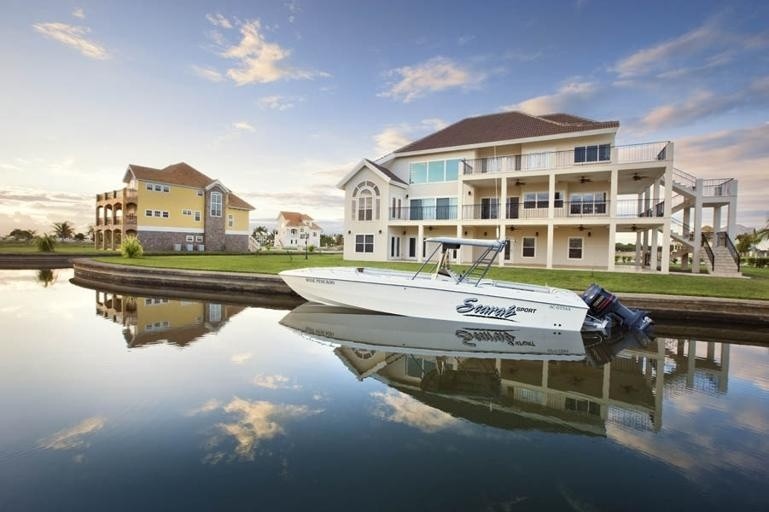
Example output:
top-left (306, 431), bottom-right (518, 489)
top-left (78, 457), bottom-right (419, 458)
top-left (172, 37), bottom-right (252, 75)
top-left (277, 235), bottom-right (660, 347)
top-left (277, 299), bottom-right (628, 365)
